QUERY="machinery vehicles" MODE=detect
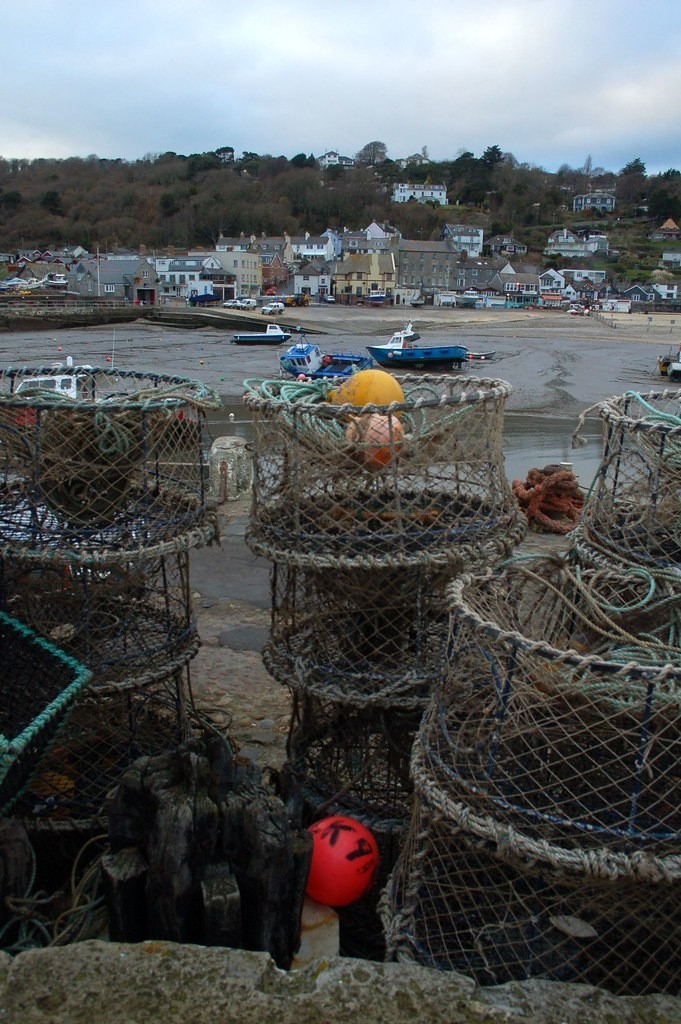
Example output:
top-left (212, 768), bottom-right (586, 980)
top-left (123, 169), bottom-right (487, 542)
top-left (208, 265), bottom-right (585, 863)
top-left (285, 293), bottom-right (309, 307)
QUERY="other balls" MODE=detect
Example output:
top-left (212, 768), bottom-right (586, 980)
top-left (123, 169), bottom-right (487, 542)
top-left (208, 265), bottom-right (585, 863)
top-left (346, 414), bottom-right (404, 471)
top-left (305, 816), bottom-right (382, 910)
top-left (331, 367), bottom-right (403, 424)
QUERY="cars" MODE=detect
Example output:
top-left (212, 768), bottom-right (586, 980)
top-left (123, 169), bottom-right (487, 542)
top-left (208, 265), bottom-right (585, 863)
top-left (261, 303), bottom-right (286, 316)
top-left (223, 300), bottom-right (240, 309)
top-left (236, 299), bottom-right (257, 310)
top-left (325, 295), bottom-right (336, 303)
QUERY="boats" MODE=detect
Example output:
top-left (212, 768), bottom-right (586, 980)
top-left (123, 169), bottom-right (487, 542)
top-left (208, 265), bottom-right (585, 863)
top-left (364, 290), bottom-right (385, 306)
top-left (466, 351), bottom-right (496, 360)
top-left (365, 320), bottom-right (469, 372)
top-left (454, 291), bottom-right (479, 304)
top-left (230, 317), bottom-right (292, 344)
top-left (15, 356), bottom-right (205, 448)
top-left (278, 323), bottom-right (373, 380)
